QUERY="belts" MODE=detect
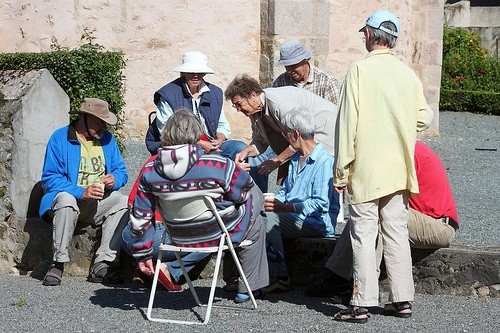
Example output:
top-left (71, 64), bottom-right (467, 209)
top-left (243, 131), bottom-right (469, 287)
top-left (440, 217), bottom-right (460, 232)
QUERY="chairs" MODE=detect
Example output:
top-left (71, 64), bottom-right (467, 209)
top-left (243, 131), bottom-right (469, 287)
top-left (145, 195), bottom-right (257, 326)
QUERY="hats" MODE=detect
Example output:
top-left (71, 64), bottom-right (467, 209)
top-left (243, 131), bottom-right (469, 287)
top-left (68, 98), bottom-right (117, 125)
top-left (173, 51), bottom-right (214, 73)
top-left (358, 11), bottom-right (400, 39)
top-left (277, 40), bottom-right (311, 66)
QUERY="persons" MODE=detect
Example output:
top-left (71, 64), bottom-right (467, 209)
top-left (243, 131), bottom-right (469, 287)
top-left (39, 98), bottom-right (128, 286)
top-left (334, 10), bottom-right (434, 324)
top-left (129, 106), bottom-right (269, 303)
top-left (308, 142), bottom-right (458, 297)
top-left (225, 39), bottom-right (342, 293)
top-left (145, 51), bottom-right (268, 194)
top-left (121, 212), bottom-right (209, 291)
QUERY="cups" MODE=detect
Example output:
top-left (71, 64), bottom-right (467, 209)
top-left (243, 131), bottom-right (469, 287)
top-left (262, 193), bottom-right (274, 204)
top-left (93, 183), bottom-right (105, 198)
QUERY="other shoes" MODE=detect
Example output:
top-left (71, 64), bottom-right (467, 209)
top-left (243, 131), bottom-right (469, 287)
top-left (234, 292), bottom-right (250, 302)
top-left (261, 277), bottom-right (290, 294)
top-left (304, 281), bottom-right (336, 296)
top-left (330, 286), bottom-right (352, 304)
top-left (225, 276), bottom-right (238, 291)
top-left (154, 265), bottom-right (182, 290)
top-left (133, 265), bottom-right (151, 285)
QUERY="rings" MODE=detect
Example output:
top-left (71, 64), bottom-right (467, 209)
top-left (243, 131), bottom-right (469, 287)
top-left (268, 169), bottom-right (270, 172)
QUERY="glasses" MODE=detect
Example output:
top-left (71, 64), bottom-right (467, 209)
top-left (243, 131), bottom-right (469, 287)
top-left (232, 97), bottom-right (246, 108)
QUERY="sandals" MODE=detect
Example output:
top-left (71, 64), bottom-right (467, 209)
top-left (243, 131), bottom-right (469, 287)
top-left (43, 264), bottom-right (64, 286)
top-left (384, 301), bottom-right (412, 317)
top-left (332, 306), bottom-right (367, 323)
top-left (88, 262), bottom-right (123, 286)
